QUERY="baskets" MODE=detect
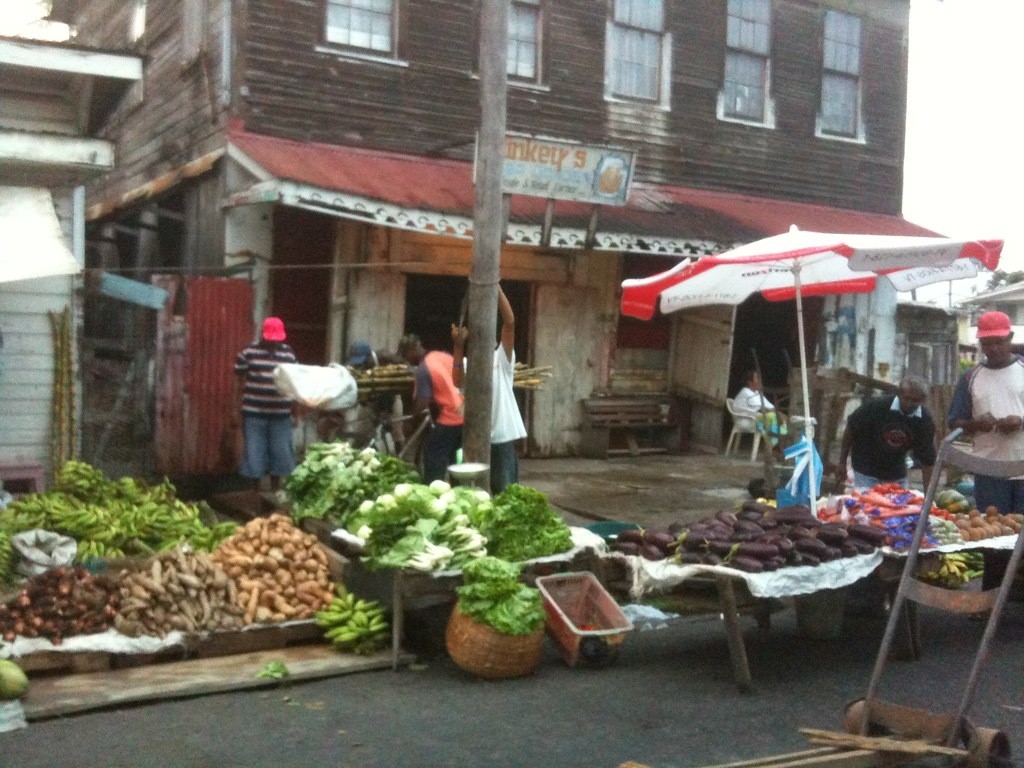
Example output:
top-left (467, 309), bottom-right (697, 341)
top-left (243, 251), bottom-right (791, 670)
top-left (445, 602), bottom-right (544, 678)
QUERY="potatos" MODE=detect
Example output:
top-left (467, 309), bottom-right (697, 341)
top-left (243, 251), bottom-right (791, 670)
top-left (945, 506), bottom-right (1023, 542)
top-left (111, 511), bottom-right (335, 639)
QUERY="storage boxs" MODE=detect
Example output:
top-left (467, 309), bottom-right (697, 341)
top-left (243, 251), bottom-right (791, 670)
top-left (534, 570), bottom-right (637, 671)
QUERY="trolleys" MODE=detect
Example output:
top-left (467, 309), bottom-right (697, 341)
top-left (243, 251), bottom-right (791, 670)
top-left (620, 429), bottom-right (1023, 767)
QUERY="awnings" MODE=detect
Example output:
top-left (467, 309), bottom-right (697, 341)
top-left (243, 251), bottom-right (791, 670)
top-left (0, 186), bottom-right (82, 283)
top-left (218, 132), bottom-right (954, 256)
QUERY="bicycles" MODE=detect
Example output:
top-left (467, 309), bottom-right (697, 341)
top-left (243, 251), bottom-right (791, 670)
top-left (320, 393), bottom-right (434, 481)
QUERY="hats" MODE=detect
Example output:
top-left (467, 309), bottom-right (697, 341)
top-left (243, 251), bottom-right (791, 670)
top-left (396, 334), bottom-right (419, 356)
top-left (262, 317), bottom-right (286, 342)
top-left (975, 312), bottom-right (1011, 338)
top-left (349, 340), bottom-right (371, 366)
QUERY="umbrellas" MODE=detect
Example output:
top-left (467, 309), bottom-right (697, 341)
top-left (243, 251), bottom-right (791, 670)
top-left (619, 224), bottom-right (1003, 521)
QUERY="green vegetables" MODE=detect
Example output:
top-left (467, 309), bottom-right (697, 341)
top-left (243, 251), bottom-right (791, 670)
top-left (350, 479), bottom-right (494, 575)
top-left (283, 439), bottom-right (421, 531)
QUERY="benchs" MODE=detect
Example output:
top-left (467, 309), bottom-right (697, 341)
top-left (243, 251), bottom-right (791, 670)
top-left (578, 398), bottom-right (691, 460)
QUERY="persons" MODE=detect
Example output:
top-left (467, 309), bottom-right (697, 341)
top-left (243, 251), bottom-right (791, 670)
top-left (730, 369), bottom-right (788, 452)
top-left (945, 311), bottom-right (1024, 608)
top-left (450, 281), bottom-right (528, 497)
top-left (344, 341), bottom-right (413, 458)
top-left (398, 333), bottom-right (464, 486)
top-left (837, 373), bottom-right (938, 502)
top-left (234, 315), bottom-right (300, 493)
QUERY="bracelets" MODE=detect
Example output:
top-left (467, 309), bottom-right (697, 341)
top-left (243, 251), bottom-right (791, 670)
top-left (453, 364), bottom-right (464, 368)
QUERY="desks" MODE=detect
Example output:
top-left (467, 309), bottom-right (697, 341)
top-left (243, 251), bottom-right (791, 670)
top-left (597, 533), bottom-right (1019, 697)
top-left (263, 489), bottom-right (602, 670)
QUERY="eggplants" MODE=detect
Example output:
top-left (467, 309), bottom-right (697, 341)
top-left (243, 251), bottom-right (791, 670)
top-left (608, 499), bottom-right (890, 572)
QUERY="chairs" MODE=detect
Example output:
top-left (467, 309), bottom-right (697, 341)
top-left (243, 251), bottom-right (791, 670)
top-left (752, 344), bottom-right (794, 411)
top-left (723, 397), bottom-right (768, 461)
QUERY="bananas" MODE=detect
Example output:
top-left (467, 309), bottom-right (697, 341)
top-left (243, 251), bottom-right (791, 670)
top-left (0, 461), bottom-right (238, 582)
top-left (312, 582), bottom-right (390, 654)
top-left (914, 551), bottom-right (984, 586)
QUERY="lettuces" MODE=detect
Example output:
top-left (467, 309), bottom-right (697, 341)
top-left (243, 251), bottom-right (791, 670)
top-left (454, 556), bottom-right (546, 636)
top-left (486, 483), bottom-right (574, 561)
